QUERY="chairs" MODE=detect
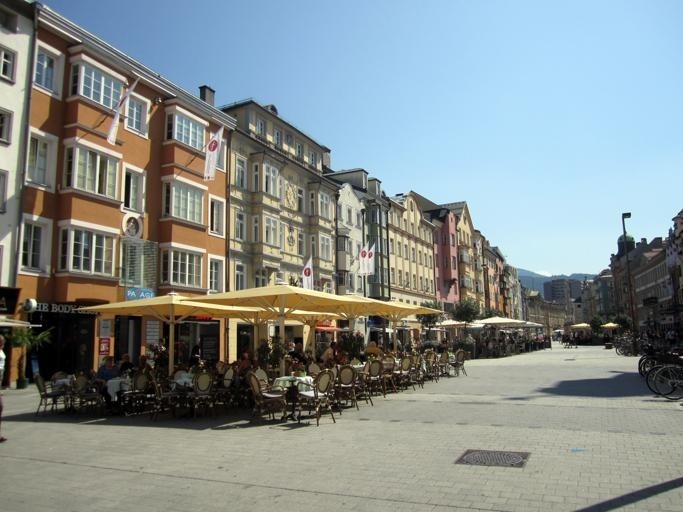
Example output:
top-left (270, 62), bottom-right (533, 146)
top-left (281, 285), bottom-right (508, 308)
top-left (32, 348), bottom-right (467, 429)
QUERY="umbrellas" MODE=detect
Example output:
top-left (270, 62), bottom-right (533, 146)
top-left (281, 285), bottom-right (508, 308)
top-left (425, 314), bottom-right (543, 332)
top-left (570, 323), bottom-right (592, 329)
top-left (599, 321), bottom-right (622, 330)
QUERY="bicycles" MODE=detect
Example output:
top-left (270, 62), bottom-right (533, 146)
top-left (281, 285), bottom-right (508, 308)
top-left (615, 334), bottom-right (682, 400)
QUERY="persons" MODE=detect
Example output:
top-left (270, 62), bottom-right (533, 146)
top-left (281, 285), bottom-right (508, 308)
top-left (95, 354), bottom-right (119, 409)
top-left (137, 354), bottom-right (153, 394)
top-left (118, 355), bottom-right (134, 375)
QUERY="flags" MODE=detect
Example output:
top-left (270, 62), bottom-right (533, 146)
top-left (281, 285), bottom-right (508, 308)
top-left (302, 254), bottom-right (313, 290)
top-left (106, 79), bottom-right (137, 146)
top-left (367, 243), bottom-right (375, 275)
top-left (358, 243), bottom-right (367, 276)
top-left (201, 126), bottom-right (223, 181)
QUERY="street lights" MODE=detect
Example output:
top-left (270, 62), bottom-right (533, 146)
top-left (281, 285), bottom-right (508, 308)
top-left (622, 212), bottom-right (638, 356)
